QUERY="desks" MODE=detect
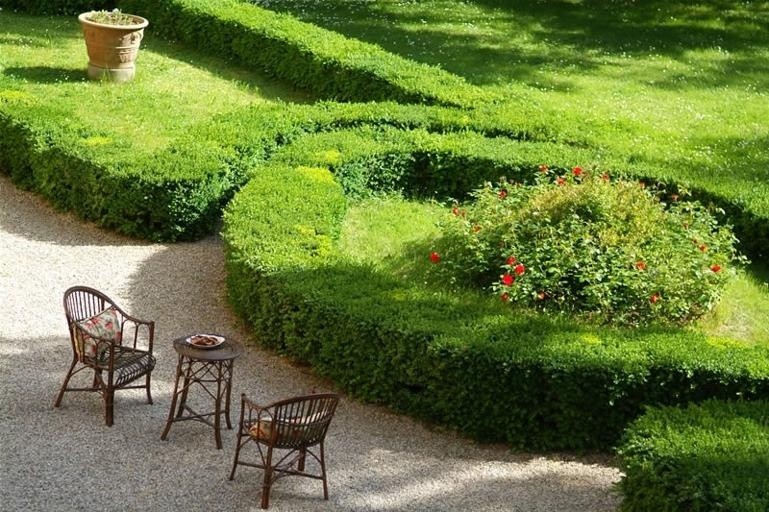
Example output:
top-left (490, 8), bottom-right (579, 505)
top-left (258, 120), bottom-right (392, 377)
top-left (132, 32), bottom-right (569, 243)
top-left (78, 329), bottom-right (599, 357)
top-left (160, 331), bottom-right (243, 450)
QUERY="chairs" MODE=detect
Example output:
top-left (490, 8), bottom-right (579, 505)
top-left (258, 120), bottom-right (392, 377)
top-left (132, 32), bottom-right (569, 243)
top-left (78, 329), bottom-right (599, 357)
top-left (228, 388), bottom-right (340, 510)
top-left (55, 285), bottom-right (156, 428)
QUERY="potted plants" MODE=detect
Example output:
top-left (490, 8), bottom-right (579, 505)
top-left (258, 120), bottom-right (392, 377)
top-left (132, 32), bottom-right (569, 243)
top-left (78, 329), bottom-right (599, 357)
top-left (78, 8), bottom-right (149, 85)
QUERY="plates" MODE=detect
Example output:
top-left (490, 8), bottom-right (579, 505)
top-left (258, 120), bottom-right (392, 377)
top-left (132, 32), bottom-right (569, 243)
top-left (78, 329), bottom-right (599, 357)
top-left (186, 334), bottom-right (225, 349)
